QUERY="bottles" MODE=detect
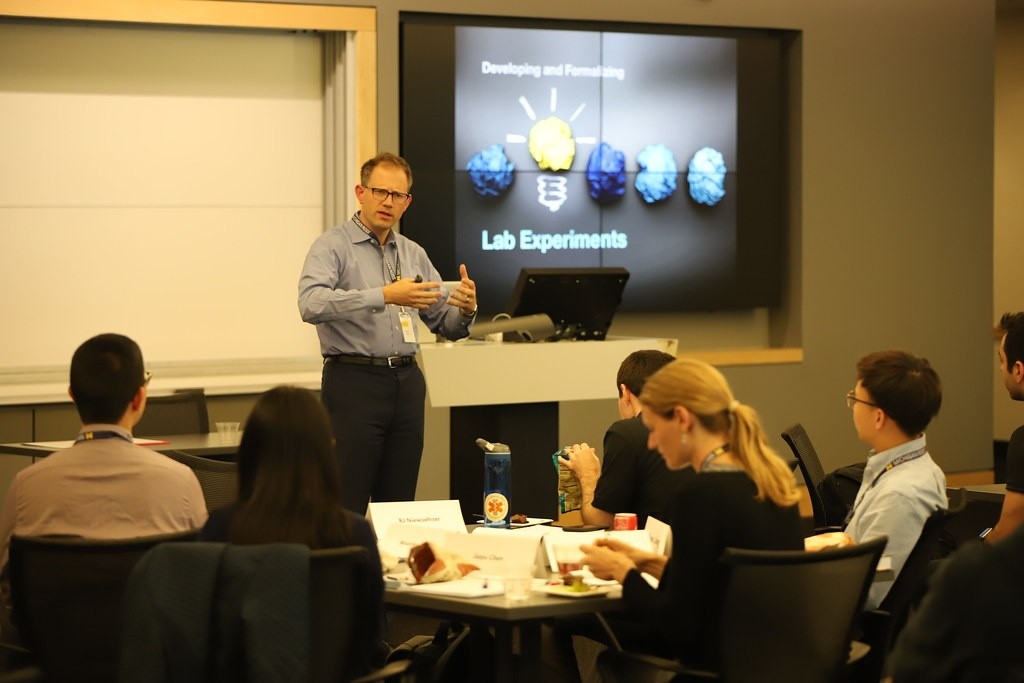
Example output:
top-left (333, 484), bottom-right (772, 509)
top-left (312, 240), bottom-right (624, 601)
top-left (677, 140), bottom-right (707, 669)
top-left (475, 439), bottom-right (511, 528)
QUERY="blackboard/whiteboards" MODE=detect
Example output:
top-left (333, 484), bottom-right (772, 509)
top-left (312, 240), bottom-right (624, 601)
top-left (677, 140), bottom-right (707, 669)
top-left (0, 16), bottom-right (335, 375)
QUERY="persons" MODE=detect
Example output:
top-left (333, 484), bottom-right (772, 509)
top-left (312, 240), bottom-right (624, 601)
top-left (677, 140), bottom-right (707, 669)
top-left (978, 311), bottom-right (1024, 548)
top-left (557, 349), bottom-right (806, 673)
top-left (0, 333), bottom-right (209, 575)
top-left (197, 386), bottom-right (386, 683)
top-left (297, 152), bottom-right (477, 519)
top-left (844, 350), bottom-right (950, 611)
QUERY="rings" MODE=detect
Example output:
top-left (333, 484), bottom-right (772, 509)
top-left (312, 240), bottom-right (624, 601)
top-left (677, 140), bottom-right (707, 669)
top-left (464, 297), bottom-right (469, 303)
top-left (567, 451), bottom-right (574, 455)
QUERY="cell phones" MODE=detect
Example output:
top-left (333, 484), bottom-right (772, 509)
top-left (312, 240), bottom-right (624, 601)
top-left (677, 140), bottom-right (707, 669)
top-left (562, 524), bottom-right (610, 532)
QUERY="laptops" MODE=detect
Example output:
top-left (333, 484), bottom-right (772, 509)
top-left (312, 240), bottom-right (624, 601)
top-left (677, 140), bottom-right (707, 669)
top-left (417, 281), bottom-right (463, 343)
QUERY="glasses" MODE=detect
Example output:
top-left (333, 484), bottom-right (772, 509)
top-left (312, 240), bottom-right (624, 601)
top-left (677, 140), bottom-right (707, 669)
top-left (361, 184), bottom-right (410, 204)
top-left (143, 372), bottom-right (153, 385)
top-left (846, 389), bottom-right (895, 420)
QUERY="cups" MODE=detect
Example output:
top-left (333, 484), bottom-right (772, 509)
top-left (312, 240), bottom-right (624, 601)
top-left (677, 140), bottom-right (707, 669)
top-left (216, 422), bottom-right (240, 445)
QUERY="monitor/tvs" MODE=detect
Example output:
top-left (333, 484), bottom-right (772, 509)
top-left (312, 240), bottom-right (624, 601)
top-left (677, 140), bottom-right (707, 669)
top-left (503, 266), bottom-right (632, 344)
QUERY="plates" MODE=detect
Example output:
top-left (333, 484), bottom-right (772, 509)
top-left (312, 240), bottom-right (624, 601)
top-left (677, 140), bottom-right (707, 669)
top-left (534, 585), bottom-right (610, 598)
top-left (477, 518), bottom-right (554, 527)
top-left (383, 573), bottom-right (417, 586)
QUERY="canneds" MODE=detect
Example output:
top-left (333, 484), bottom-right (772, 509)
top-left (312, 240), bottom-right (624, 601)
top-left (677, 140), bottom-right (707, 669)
top-left (614, 513), bottom-right (638, 531)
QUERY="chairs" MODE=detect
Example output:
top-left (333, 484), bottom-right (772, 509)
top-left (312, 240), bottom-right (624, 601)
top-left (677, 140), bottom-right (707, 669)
top-left (0, 387), bottom-right (968, 683)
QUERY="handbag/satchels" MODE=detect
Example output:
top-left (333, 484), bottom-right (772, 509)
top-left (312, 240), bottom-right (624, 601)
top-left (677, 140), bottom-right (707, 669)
top-left (414, 616), bottom-right (496, 683)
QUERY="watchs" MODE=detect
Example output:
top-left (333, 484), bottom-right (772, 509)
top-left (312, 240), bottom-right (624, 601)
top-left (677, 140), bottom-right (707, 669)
top-left (460, 304), bottom-right (478, 318)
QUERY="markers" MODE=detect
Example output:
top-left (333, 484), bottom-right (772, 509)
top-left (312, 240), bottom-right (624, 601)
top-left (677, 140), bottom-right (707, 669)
top-left (414, 275), bottom-right (423, 283)
top-left (174, 388), bottom-right (205, 393)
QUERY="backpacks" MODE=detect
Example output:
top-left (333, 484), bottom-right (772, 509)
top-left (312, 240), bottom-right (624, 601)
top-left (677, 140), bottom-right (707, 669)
top-left (817, 462), bottom-right (867, 526)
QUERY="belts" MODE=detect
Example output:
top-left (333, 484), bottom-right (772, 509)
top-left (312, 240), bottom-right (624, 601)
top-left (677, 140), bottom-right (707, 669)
top-left (325, 355), bottom-right (416, 370)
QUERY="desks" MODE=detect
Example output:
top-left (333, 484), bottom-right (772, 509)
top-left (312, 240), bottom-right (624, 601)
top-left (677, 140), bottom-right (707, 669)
top-left (367, 523), bottom-right (663, 683)
top-left (0, 430), bottom-right (245, 458)
top-left (944, 484), bottom-right (1006, 502)
